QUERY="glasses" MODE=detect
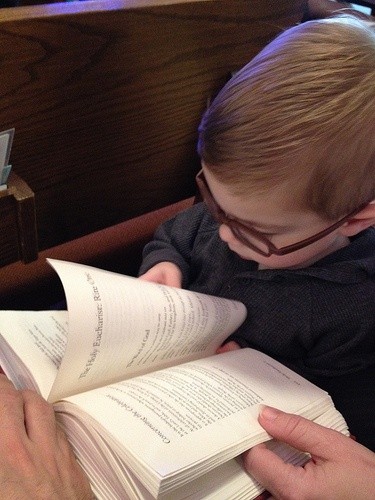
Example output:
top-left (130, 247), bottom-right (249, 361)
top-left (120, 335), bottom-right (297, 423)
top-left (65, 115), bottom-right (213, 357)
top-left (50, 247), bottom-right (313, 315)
top-left (194, 171), bottom-right (369, 256)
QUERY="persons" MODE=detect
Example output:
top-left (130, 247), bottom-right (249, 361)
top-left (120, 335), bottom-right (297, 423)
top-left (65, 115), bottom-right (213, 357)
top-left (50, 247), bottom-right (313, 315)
top-left (134, 7), bottom-right (375, 453)
top-left (0, 371), bottom-right (96, 500)
top-left (243, 404), bottom-right (375, 500)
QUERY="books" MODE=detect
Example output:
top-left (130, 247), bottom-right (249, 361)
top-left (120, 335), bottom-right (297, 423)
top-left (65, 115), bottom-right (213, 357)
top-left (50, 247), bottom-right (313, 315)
top-left (0, 258), bottom-right (356, 500)
